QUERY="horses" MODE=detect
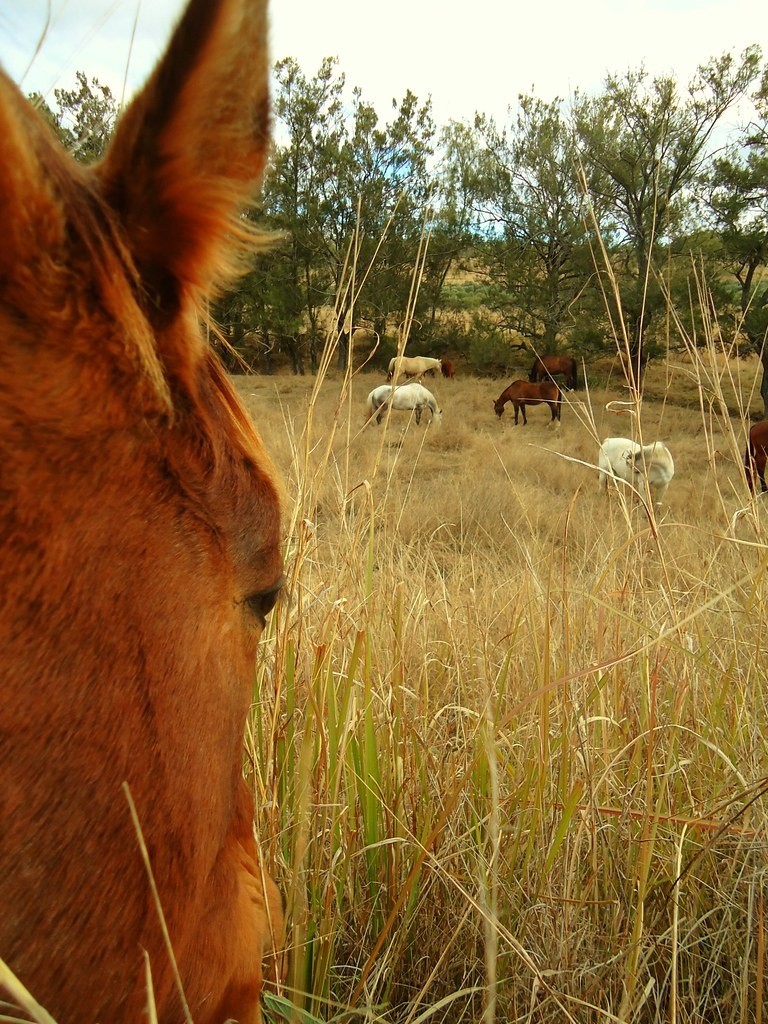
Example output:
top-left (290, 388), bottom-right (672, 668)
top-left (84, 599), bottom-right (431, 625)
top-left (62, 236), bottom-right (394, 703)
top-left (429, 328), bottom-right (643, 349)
top-left (491, 380), bottom-right (562, 425)
top-left (527, 355), bottom-right (578, 391)
top-left (365, 383), bottom-right (442, 426)
top-left (386, 355), bottom-right (442, 384)
top-left (443, 360), bottom-right (456, 378)
top-left (597, 438), bottom-right (675, 515)
top-left (1, 2), bottom-right (297, 1024)
top-left (744, 420), bottom-right (768, 497)
